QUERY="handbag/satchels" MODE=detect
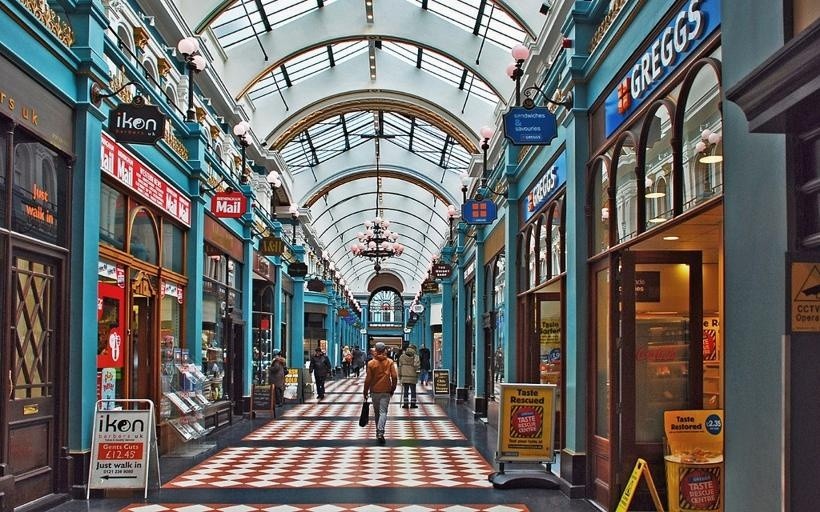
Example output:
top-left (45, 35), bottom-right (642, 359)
top-left (360, 403), bottom-right (369, 427)
top-left (284, 365), bottom-right (287, 375)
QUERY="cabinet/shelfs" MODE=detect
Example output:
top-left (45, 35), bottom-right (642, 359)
top-left (643, 314), bottom-right (722, 412)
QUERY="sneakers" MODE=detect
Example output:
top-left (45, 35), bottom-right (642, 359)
top-left (377, 432), bottom-right (385, 443)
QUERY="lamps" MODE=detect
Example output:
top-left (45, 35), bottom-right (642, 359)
top-left (350, 132), bottom-right (404, 279)
top-left (504, 46), bottom-right (529, 106)
top-left (233, 120), bottom-right (253, 176)
top-left (265, 171), bottom-right (362, 316)
top-left (694, 124), bottom-right (722, 196)
top-left (477, 128), bottom-right (497, 183)
top-left (408, 172), bottom-right (470, 316)
top-left (178, 38), bottom-right (206, 122)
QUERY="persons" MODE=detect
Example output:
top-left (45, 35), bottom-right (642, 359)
top-left (363, 341), bottom-right (398, 445)
top-left (309, 348), bottom-right (332, 399)
top-left (418, 343), bottom-right (431, 385)
top-left (267, 349), bottom-right (289, 408)
top-left (398, 345), bottom-right (421, 409)
top-left (341, 345), bottom-right (403, 378)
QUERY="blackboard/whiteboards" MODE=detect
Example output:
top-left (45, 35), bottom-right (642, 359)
top-left (432, 369), bottom-right (450, 397)
top-left (250, 384), bottom-right (274, 413)
top-left (283, 368), bottom-right (302, 400)
